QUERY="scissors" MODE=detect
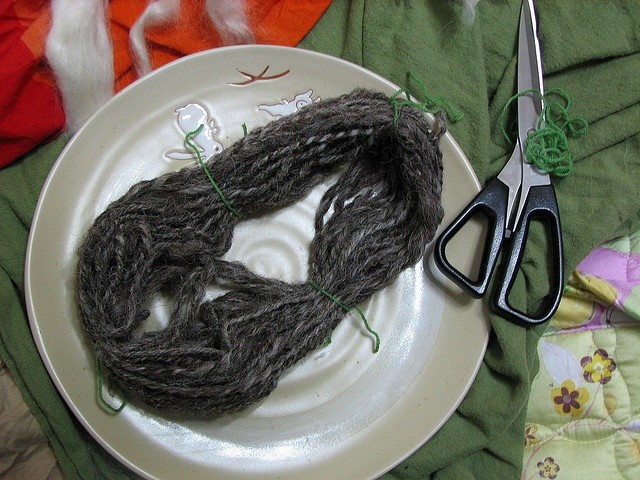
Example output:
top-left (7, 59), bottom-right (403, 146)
top-left (434, 1), bottom-right (564, 326)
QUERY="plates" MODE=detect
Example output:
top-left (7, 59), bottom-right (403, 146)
top-left (22, 42), bottom-right (498, 475)
